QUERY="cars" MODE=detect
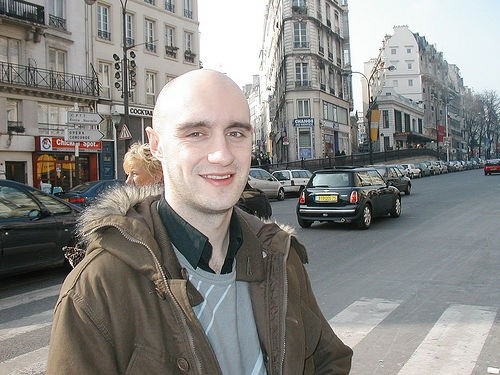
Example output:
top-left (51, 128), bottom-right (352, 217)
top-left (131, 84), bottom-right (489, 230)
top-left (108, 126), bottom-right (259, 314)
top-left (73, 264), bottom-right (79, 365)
top-left (376, 166), bottom-right (411, 195)
top-left (296, 166), bottom-right (401, 231)
top-left (0, 178), bottom-right (85, 279)
top-left (247, 168), bottom-right (285, 201)
top-left (484, 161), bottom-right (500, 175)
top-left (272, 169), bottom-right (314, 198)
top-left (53, 178), bottom-right (125, 210)
top-left (401, 157), bottom-right (500, 179)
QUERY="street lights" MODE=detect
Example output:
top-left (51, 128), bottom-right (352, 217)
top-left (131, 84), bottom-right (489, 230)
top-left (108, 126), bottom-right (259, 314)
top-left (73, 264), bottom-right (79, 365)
top-left (340, 66), bottom-right (397, 165)
top-left (113, 51), bottom-right (136, 153)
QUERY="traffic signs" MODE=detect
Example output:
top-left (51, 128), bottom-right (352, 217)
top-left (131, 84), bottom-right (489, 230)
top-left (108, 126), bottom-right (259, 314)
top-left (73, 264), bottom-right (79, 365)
top-left (64, 128), bottom-right (104, 142)
top-left (65, 111), bottom-right (105, 126)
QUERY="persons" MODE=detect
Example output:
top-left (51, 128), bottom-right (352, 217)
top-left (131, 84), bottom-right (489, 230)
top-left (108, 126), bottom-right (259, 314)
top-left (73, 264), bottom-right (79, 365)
top-left (123, 140), bottom-right (164, 186)
top-left (46, 69), bottom-right (353, 375)
